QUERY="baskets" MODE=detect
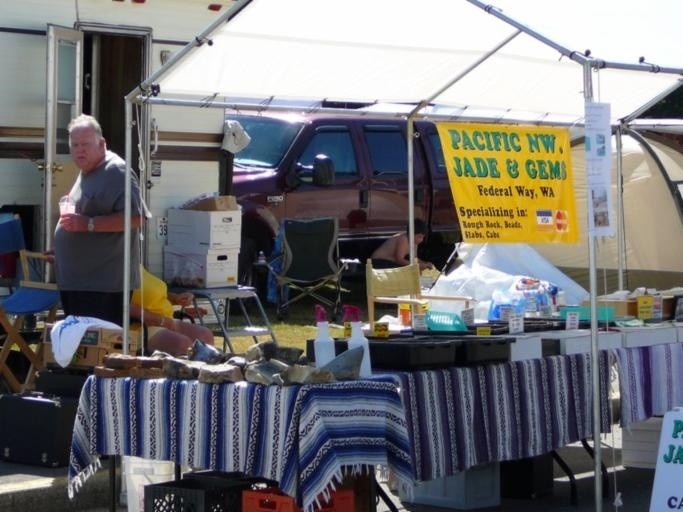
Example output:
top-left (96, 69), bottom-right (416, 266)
top-left (145, 471), bottom-right (356, 512)
top-left (0, 390), bottom-right (78, 469)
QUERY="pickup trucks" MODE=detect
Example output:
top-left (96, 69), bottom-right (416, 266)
top-left (222, 103), bottom-right (587, 314)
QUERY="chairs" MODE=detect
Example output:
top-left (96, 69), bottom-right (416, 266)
top-left (365, 258), bottom-right (469, 334)
top-left (0, 250), bottom-right (60, 395)
top-left (268, 217), bottom-right (351, 320)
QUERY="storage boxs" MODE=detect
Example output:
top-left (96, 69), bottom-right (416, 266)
top-left (163, 246), bottom-right (240, 289)
top-left (307, 285), bottom-right (683, 371)
top-left (0, 324), bottom-right (139, 468)
top-left (167, 207), bottom-right (242, 249)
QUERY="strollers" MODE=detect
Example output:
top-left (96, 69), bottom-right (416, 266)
top-left (253, 217), bottom-right (349, 319)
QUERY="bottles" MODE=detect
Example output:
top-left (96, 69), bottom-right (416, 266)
top-left (257, 250), bottom-right (266, 263)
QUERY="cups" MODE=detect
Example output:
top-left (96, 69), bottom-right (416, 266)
top-left (58, 201), bottom-right (76, 220)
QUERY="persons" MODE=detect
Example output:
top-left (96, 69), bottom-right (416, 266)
top-left (51, 112), bottom-right (152, 371)
top-left (130, 261), bottom-right (215, 356)
top-left (367, 217), bottom-right (433, 277)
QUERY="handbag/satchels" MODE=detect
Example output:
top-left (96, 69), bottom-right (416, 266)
top-left (37, 363), bottom-right (90, 390)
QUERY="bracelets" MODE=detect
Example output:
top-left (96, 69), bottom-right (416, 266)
top-left (159, 313), bottom-right (165, 326)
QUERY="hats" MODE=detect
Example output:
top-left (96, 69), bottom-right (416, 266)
top-left (222, 120), bottom-right (251, 154)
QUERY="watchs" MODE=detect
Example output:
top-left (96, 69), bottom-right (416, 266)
top-left (87, 218), bottom-right (96, 234)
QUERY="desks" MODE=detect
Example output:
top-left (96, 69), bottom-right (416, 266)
top-left (364, 341), bottom-right (683, 511)
top-left (64, 372), bottom-right (416, 512)
top-left (172, 289), bottom-right (276, 352)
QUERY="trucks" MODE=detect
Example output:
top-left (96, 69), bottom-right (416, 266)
top-left (0, 1), bottom-right (253, 339)
